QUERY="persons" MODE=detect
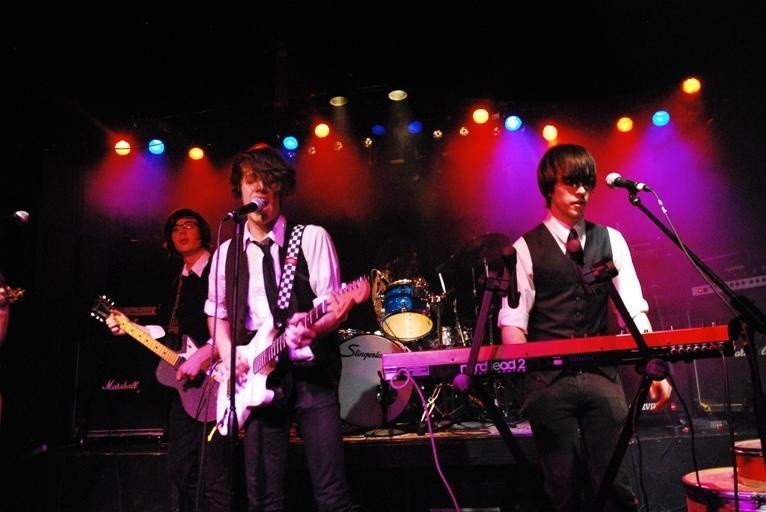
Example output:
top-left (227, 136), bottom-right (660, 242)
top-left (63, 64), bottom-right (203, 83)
top-left (105, 210), bottom-right (236, 512)
top-left (496, 145), bottom-right (673, 512)
top-left (203, 148), bottom-right (352, 510)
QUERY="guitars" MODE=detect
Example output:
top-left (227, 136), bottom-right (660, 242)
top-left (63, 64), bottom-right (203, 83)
top-left (217, 274), bottom-right (371, 435)
top-left (89, 292), bottom-right (217, 423)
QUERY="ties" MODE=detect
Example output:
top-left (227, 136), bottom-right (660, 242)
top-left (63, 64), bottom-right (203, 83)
top-left (567, 228), bottom-right (584, 267)
top-left (251, 239), bottom-right (280, 328)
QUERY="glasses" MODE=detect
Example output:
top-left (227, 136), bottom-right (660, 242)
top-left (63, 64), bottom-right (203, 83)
top-left (171, 222), bottom-right (197, 233)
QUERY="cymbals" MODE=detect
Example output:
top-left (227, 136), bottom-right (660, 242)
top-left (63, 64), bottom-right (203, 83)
top-left (460, 232), bottom-right (512, 274)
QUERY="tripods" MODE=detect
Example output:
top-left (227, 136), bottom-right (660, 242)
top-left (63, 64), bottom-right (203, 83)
top-left (417, 383), bottom-right (494, 435)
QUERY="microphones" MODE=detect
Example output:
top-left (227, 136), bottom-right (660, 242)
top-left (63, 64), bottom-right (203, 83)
top-left (605, 172), bottom-right (651, 192)
top-left (223, 197), bottom-right (265, 221)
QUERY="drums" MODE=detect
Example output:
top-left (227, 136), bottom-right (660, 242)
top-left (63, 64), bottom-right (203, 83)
top-left (429, 326), bottom-right (460, 349)
top-left (681, 465), bottom-right (765, 512)
top-left (460, 327), bottom-right (474, 348)
top-left (338, 329), bottom-right (416, 427)
top-left (732, 439), bottom-right (765, 486)
top-left (376, 280), bottom-right (433, 341)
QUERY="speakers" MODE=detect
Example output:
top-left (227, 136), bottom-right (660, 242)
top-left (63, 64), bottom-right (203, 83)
top-left (69, 331), bottom-right (178, 457)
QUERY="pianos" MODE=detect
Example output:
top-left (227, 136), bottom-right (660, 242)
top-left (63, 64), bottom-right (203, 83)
top-left (381, 324), bottom-right (730, 382)
